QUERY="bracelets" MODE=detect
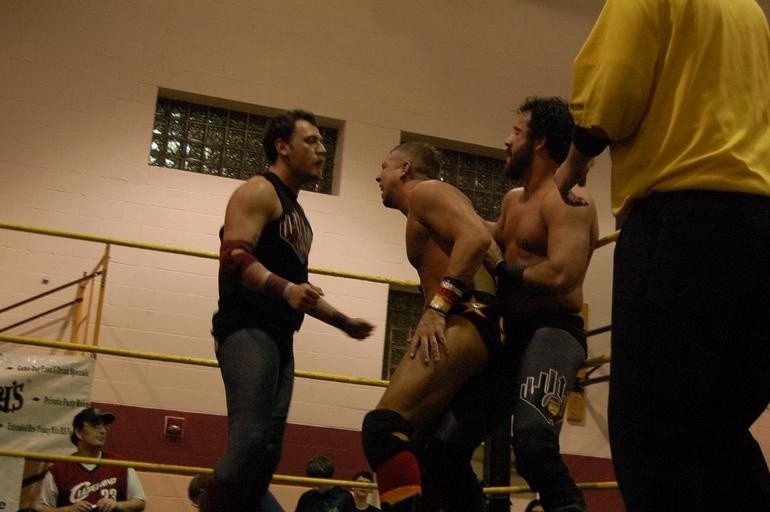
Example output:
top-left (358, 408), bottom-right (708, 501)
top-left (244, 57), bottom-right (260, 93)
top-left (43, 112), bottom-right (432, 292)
top-left (263, 271), bottom-right (296, 304)
top-left (427, 274), bottom-right (468, 320)
top-left (332, 310), bottom-right (348, 328)
top-left (494, 260), bottom-right (529, 293)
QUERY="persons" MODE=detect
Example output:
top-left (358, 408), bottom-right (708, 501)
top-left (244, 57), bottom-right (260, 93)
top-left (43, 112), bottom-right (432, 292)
top-left (551, 0), bottom-right (770, 512)
top-left (209, 108), bottom-right (376, 510)
top-left (424, 96), bottom-right (599, 512)
top-left (31, 406), bottom-right (148, 511)
top-left (360, 141), bottom-right (504, 511)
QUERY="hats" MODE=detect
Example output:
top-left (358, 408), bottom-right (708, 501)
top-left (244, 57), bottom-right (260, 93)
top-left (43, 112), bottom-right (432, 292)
top-left (73, 408), bottom-right (116, 426)
